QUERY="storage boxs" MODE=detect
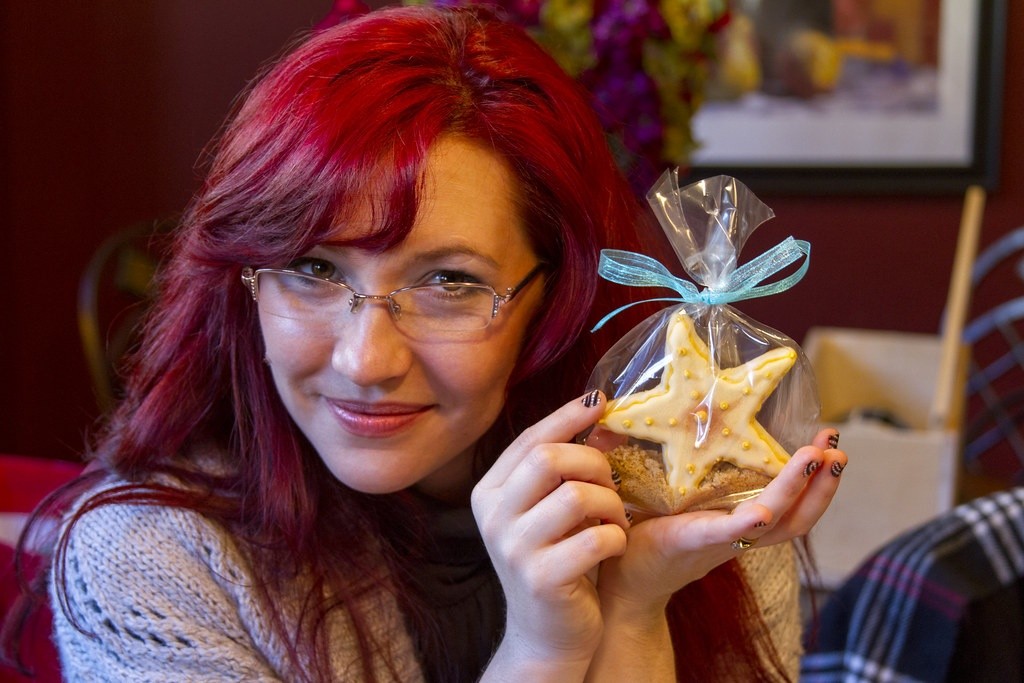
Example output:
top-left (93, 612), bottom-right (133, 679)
top-left (774, 323), bottom-right (973, 595)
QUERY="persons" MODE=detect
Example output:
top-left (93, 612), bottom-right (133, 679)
top-left (50, 6), bottom-right (848, 683)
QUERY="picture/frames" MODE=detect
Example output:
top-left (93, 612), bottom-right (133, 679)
top-left (682, 0), bottom-right (1010, 198)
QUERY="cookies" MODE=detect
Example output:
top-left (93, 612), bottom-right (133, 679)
top-left (598, 309), bottom-right (797, 509)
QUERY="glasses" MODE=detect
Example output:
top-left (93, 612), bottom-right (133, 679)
top-left (242, 259), bottom-right (544, 331)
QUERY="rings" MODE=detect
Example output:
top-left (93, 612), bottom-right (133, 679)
top-left (735, 538), bottom-right (755, 556)
